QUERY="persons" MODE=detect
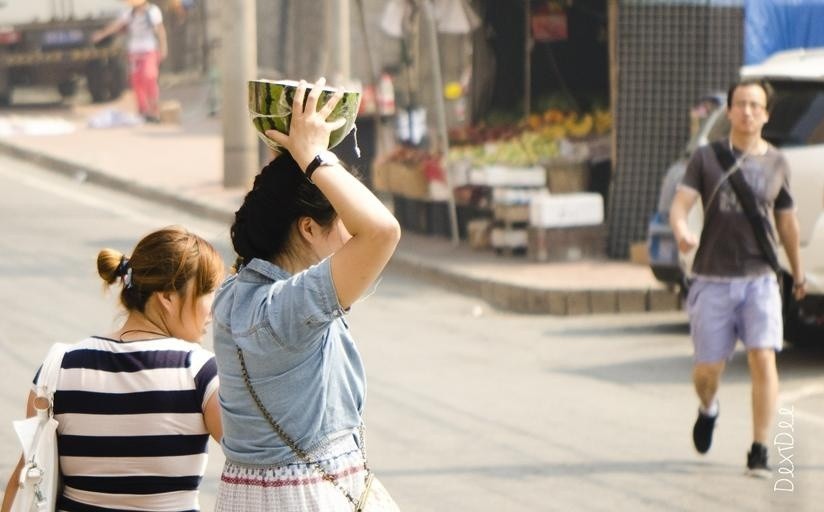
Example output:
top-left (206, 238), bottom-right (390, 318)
top-left (92, 0), bottom-right (168, 124)
top-left (162, 0), bottom-right (195, 75)
top-left (2, 224), bottom-right (226, 512)
top-left (665, 73), bottom-right (806, 479)
top-left (204, 73), bottom-right (404, 512)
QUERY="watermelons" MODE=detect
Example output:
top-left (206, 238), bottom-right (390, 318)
top-left (248, 80), bottom-right (360, 151)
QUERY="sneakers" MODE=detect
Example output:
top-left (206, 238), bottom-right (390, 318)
top-left (694, 400), bottom-right (719, 453)
top-left (747, 443), bottom-right (772, 478)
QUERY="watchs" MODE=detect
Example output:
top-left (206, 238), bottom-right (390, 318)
top-left (304, 150), bottom-right (341, 183)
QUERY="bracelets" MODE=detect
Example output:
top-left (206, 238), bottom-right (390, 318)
top-left (792, 278), bottom-right (806, 288)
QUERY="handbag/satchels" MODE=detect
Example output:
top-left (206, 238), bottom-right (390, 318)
top-left (10, 416), bottom-right (61, 512)
top-left (355, 474), bottom-right (400, 512)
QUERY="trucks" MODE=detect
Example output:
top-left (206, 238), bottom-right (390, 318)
top-left (0, 0), bottom-right (137, 107)
